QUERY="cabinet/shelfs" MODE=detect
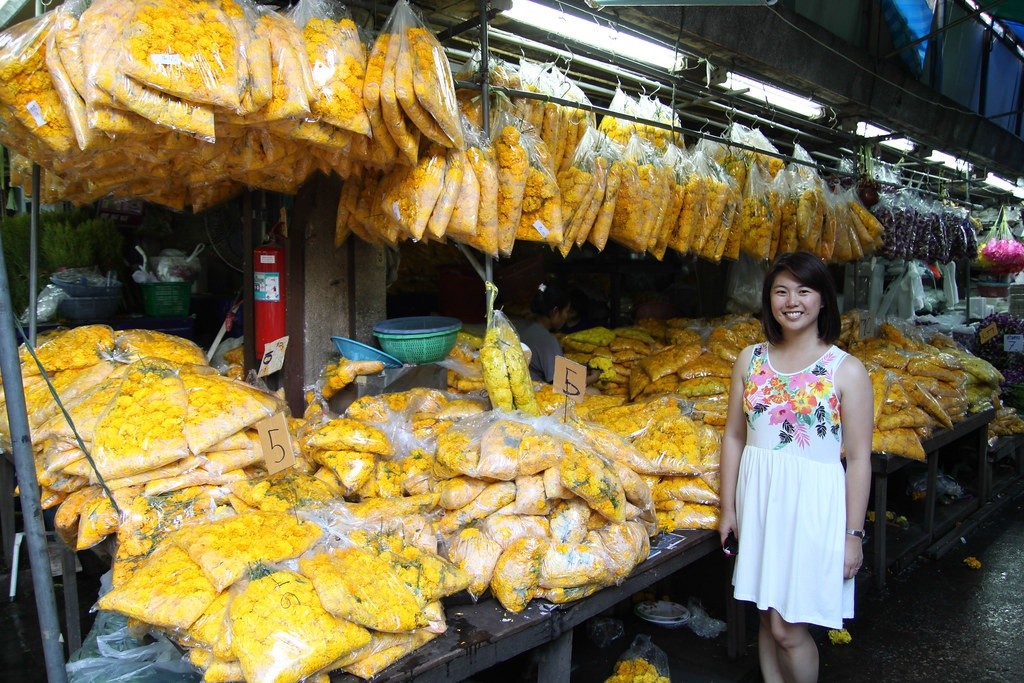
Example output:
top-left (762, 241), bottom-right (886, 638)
top-left (840, 408), bottom-right (995, 591)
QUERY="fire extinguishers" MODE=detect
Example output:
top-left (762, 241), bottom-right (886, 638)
top-left (253, 222), bottom-right (289, 362)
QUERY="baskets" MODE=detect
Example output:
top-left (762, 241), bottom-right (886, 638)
top-left (372, 315), bottom-right (463, 365)
top-left (143, 282), bottom-right (192, 318)
top-left (48, 274), bottom-right (122, 322)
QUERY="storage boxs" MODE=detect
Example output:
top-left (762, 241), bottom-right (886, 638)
top-left (52, 274), bottom-right (192, 327)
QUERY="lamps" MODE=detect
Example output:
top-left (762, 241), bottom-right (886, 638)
top-left (496, 0), bottom-right (1024, 202)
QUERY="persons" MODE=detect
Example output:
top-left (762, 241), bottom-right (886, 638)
top-left (520, 282), bottom-right (601, 395)
top-left (719, 252), bottom-right (874, 683)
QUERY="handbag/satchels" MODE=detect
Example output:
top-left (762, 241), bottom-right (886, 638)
top-left (969, 206), bottom-right (1024, 273)
top-left (131, 243), bottom-right (205, 294)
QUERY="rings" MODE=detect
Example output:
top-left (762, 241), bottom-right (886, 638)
top-left (855, 568), bottom-right (859, 569)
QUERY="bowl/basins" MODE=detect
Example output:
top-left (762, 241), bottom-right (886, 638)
top-left (57, 296), bottom-right (119, 320)
top-left (50, 275), bottom-right (123, 296)
top-left (977, 282), bottom-right (1010, 297)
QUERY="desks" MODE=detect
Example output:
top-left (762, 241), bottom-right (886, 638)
top-left (330, 470), bottom-right (749, 683)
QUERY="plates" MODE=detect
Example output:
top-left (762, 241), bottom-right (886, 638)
top-left (635, 601), bottom-right (690, 629)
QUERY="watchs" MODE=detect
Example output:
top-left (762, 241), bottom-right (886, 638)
top-left (846, 529), bottom-right (865, 538)
